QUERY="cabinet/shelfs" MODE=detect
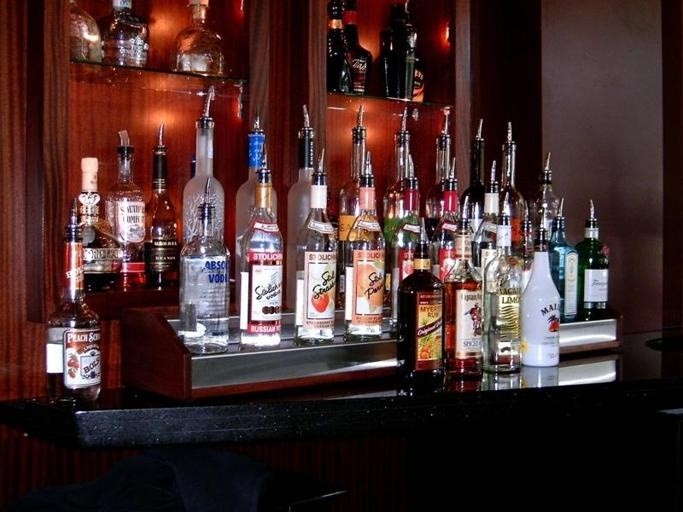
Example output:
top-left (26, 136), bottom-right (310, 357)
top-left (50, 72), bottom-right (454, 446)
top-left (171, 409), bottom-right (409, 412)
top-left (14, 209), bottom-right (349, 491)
top-left (30, 0), bottom-right (626, 399)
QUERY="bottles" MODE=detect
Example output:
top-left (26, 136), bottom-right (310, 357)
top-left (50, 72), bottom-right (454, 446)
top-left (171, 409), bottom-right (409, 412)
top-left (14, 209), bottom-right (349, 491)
top-left (47, 194), bottom-right (103, 399)
top-left (293, 143), bottom-right (337, 343)
top-left (76, 146), bottom-right (148, 300)
top-left (169, 0), bottom-right (228, 77)
top-left (284, 130), bottom-right (329, 311)
top-left (238, 130), bottom-right (285, 346)
top-left (327, 0), bottom-right (427, 103)
top-left (97, 0), bottom-right (149, 67)
top-left (70, 0), bottom-right (101, 64)
top-left (149, 146), bottom-right (180, 294)
top-left (179, 118), bottom-right (227, 247)
top-left (336, 126), bottom-right (615, 398)
top-left (236, 135), bottom-right (279, 236)
top-left (179, 175), bottom-right (231, 356)
